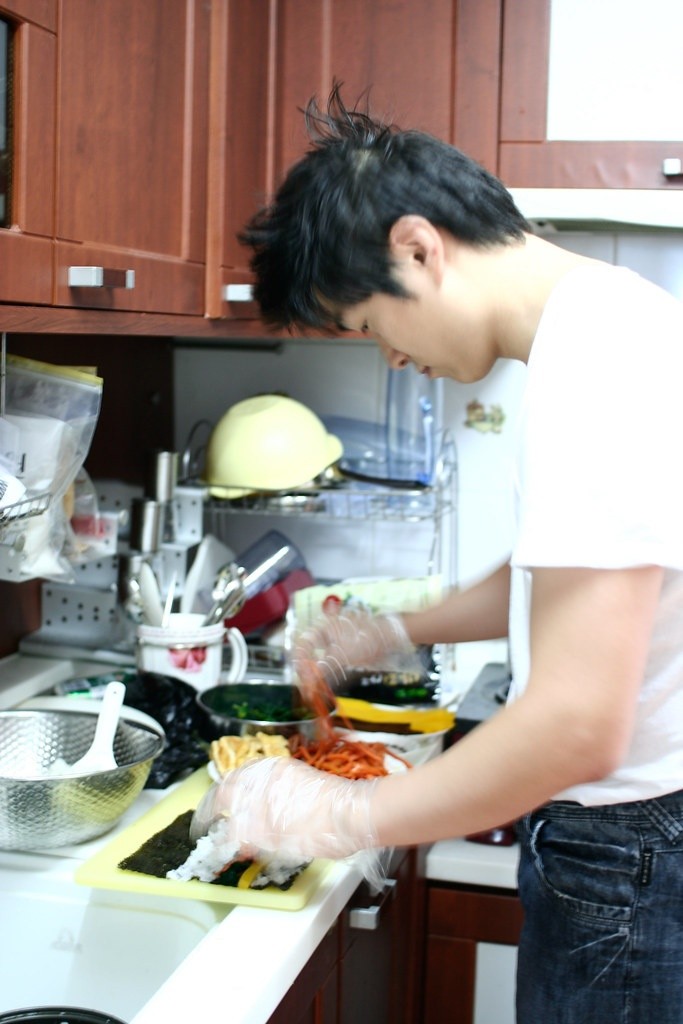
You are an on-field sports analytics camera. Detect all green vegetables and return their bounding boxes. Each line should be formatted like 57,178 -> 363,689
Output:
231,700 -> 316,721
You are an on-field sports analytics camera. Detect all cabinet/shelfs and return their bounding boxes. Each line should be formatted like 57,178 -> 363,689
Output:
417,836 -> 521,1024
0,0 -> 503,341
0,653 -> 457,1024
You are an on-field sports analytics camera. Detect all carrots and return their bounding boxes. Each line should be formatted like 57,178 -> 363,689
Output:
288,659 -> 412,781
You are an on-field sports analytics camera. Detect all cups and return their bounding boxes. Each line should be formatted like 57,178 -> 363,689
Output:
138,612 -> 247,691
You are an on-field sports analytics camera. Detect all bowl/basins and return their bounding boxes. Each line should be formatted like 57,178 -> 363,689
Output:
203,393 -> 342,499
195,682 -> 337,737
0,709 -> 167,851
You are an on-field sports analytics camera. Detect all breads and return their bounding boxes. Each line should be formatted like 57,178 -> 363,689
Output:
211,731 -> 290,781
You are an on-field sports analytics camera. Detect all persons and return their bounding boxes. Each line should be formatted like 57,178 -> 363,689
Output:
188,74 -> 683,1024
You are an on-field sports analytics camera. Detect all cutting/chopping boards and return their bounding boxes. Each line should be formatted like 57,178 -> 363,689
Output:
78,764 -> 334,911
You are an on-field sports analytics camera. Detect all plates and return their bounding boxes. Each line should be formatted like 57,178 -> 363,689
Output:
334,704 -> 449,748
